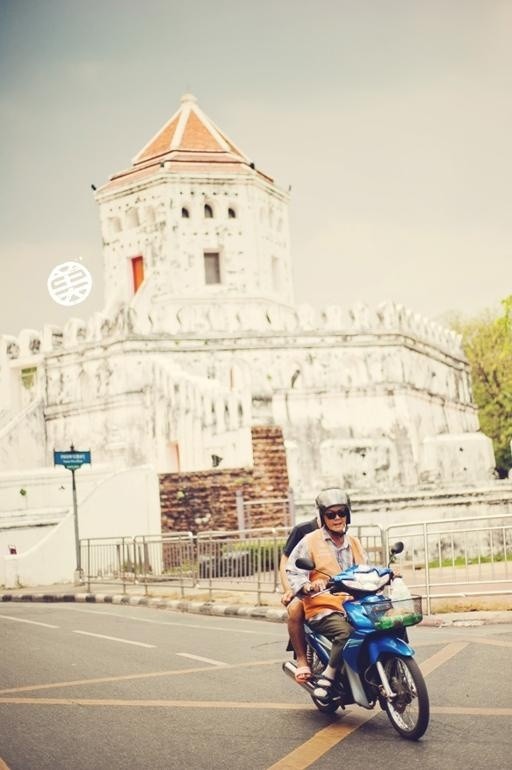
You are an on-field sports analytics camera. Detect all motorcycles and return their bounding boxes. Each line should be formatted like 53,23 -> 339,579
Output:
282,539 -> 436,742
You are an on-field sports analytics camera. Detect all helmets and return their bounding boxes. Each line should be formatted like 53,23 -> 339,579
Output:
315,487 -> 350,528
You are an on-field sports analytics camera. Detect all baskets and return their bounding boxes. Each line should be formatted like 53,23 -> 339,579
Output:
361,595 -> 422,631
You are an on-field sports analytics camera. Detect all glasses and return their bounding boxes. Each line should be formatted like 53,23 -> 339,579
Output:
326,511 -> 346,519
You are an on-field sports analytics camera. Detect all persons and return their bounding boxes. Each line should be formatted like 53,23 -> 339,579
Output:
282,487 -> 400,704
278,509 -> 327,684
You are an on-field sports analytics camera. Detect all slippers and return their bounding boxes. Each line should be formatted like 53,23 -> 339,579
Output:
295,667 -> 312,683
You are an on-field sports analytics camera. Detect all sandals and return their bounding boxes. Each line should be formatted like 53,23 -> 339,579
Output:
311,675 -> 334,699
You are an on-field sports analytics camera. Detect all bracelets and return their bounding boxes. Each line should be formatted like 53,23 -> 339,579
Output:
306,585 -> 311,595
